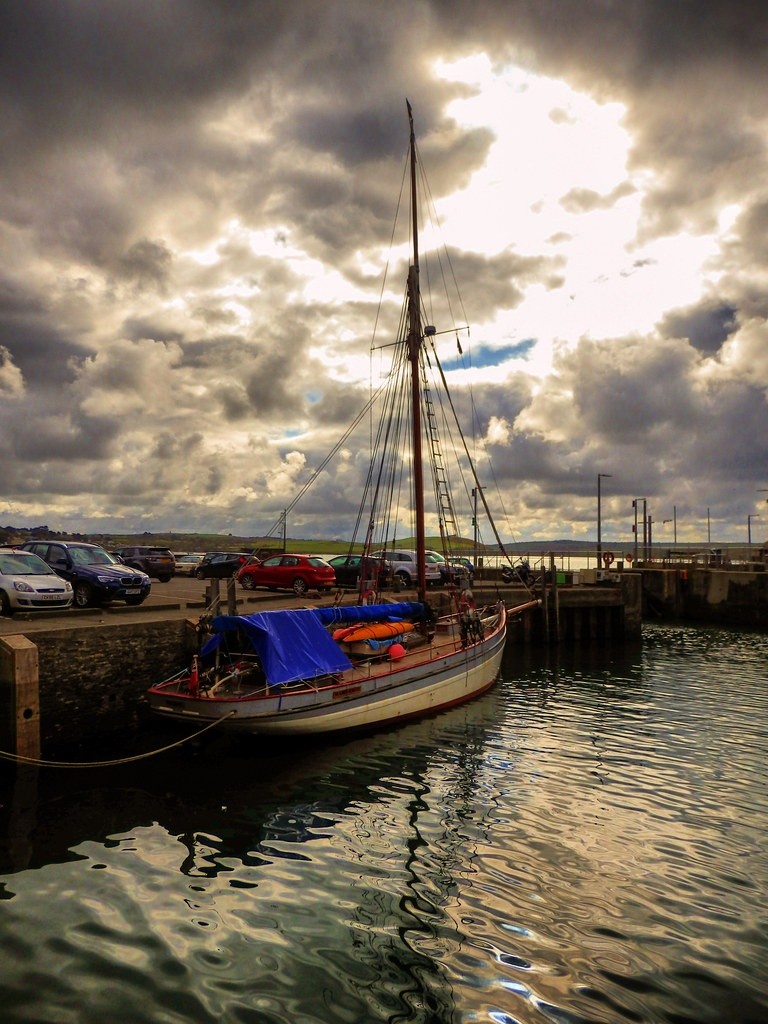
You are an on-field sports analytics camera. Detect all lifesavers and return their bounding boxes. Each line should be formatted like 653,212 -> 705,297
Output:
603,552 -> 614,564
363,590 -> 376,606
461,589 -> 475,615
626,554 -> 632,562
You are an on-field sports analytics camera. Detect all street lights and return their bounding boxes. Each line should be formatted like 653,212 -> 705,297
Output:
633,497 -> 648,568
747,513 -> 760,545
596,472 -> 613,567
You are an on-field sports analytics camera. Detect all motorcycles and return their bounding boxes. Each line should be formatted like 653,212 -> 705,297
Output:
500,556 -> 535,584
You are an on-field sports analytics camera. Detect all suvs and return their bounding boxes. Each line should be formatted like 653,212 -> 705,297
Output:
113,546 -> 176,583
21,540 -> 152,608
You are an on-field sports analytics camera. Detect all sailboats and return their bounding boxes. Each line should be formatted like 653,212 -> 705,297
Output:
130,97 -> 550,736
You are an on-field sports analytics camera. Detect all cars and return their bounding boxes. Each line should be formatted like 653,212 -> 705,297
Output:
323,555 -> 394,592
235,553 -> 336,593
174,551 -> 260,579
0,548 -> 74,617
367,547 -> 476,587
